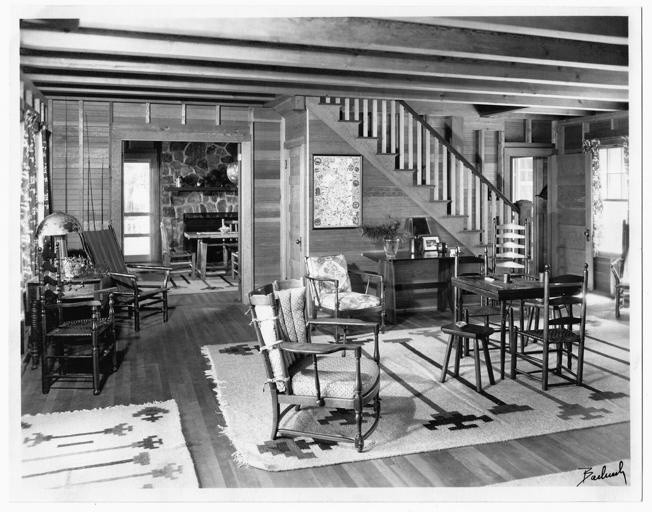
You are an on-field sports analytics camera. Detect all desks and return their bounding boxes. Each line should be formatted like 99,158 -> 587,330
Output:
361,250 -> 476,323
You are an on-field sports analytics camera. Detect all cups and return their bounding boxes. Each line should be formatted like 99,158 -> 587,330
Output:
437,242 -> 448,253
384,239 -> 399,259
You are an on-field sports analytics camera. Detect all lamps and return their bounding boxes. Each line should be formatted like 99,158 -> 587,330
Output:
36,213 -> 82,258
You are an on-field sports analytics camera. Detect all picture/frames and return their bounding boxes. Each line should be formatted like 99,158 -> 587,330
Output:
313,153 -> 363,229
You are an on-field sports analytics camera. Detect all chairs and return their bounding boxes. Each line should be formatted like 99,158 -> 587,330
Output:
76,225 -> 172,331
305,253 -> 385,357
609,220 -> 632,321
511,263 -> 589,391
231,252 -> 240,280
222,219 -> 239,265
245,278 -> 379,453
160,218 -> 195,288
38,242 -> 117,395
455,243 -> 514,356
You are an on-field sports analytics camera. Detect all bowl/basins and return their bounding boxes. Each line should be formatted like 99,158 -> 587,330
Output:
218,225 -> 231,234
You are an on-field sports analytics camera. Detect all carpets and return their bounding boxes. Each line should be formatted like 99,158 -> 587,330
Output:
202,315 -> 630,472
137,276 -> 239,294
21,399 -> 200,489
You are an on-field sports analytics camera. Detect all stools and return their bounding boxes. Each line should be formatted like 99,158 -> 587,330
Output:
524,301 -> 567,347
439,322 -> 496,392
